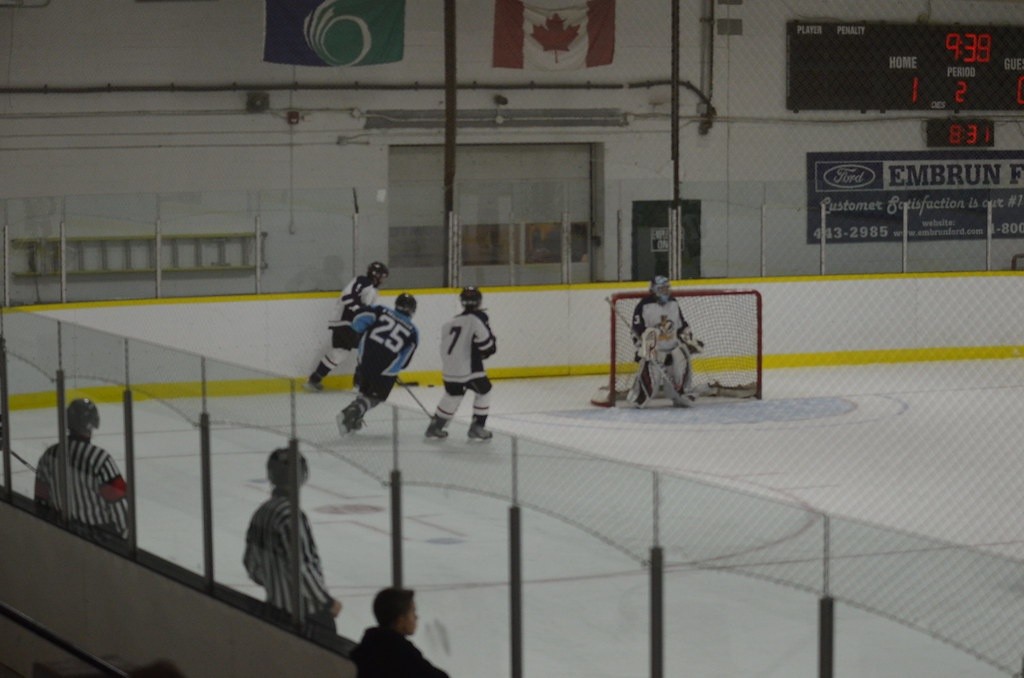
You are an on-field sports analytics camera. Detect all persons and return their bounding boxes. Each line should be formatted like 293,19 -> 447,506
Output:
35,399 -> 128,538
304,260 -> 389,392
627,275 -> 703,411
337,293 -> 418,436
245,448 -> 339,628
350,588 -> 448,678
424,286 -> 496,439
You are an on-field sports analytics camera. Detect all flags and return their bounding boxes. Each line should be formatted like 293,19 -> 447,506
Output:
267,0 -> 402,66
492,0 -> 616,69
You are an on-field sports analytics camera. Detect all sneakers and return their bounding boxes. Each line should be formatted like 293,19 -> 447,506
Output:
301,371 -> 323,391
466,414 -> 493,444
423,413 -> 448,443
335,392 -> 371,437
351,383 -> 360,394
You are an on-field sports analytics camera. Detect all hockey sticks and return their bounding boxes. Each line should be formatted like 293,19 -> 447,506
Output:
397,377 -> 434,418
395,375 -> 420,388
604,295 -> 696,408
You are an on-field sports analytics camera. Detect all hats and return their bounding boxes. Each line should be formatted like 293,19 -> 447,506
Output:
651,275 -> 673,291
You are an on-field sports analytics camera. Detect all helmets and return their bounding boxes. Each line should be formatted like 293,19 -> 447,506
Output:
395,293 -> 417,315
368,262 -> 389,287
67,398 -> 100,430
267,449 -> 308,486
460,287 -> 482,306
